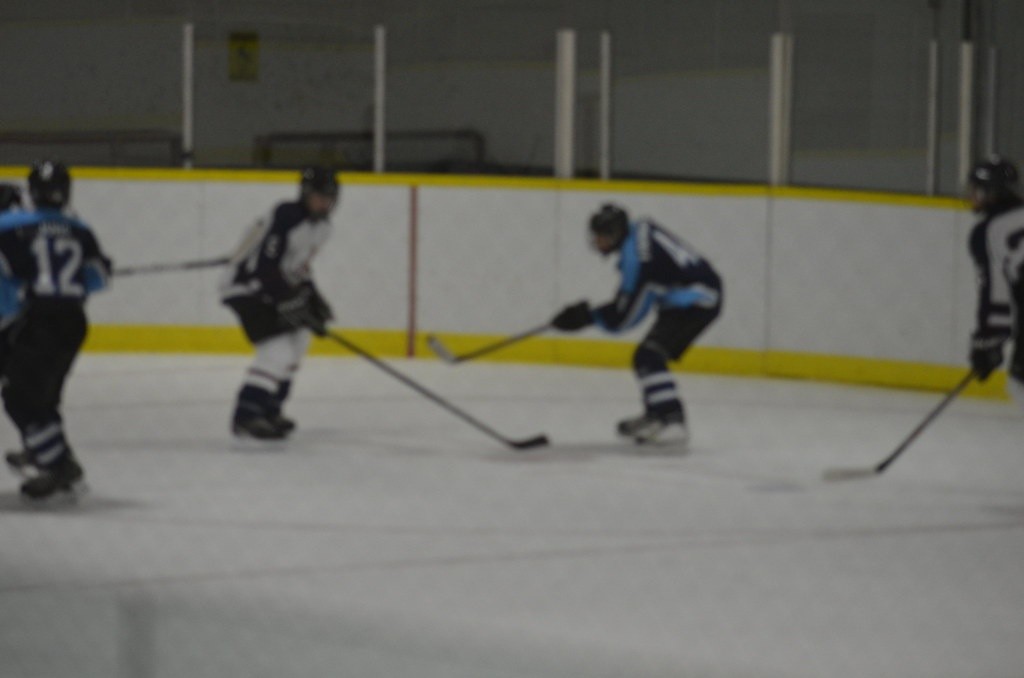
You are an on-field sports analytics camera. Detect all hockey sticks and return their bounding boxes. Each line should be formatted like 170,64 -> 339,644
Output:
424,321 -> 554,364
820,367 -> 978,481
326,332 -> 549,449
112,213 -> 275,278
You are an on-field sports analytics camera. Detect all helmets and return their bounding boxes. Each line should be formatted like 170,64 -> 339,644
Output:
965,158 -> 1019,216
299,168 -> 340,226
588,204 -> 630,255
28,154 -> 70,208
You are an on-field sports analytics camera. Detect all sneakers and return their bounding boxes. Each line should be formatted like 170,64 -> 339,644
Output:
617,382 -> 674,441
635,400 -> 687,453
258,385 -> 295,435
7,450 -> 45,477
16,462 -> 78,509
20,447 -> 92,493
232,385 -> 278,447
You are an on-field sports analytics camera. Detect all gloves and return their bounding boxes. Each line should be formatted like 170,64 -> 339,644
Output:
552,301 -> 594,333
596,290 -> 634,331
273,286 -> 326,340
970,325 -> 1010,381
295,280 -> 333,321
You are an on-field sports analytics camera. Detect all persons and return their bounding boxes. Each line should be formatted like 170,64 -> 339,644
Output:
552,203 -> 724,447
963,150 -> 1024,388
223,158 -> 340,442
1,160 -> 117,498
